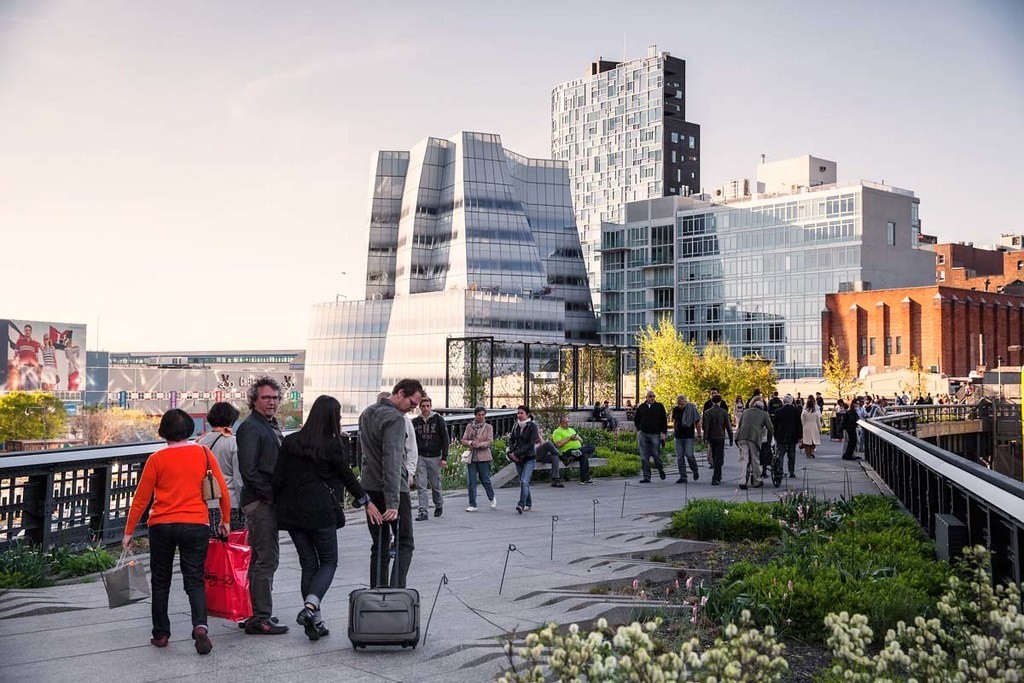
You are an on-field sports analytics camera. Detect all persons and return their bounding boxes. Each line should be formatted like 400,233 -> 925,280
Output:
593,385 -> 960,490
196,401 -> 247,540
375,391 -> 419,559
552,415 -> 596,485
13,324 -> 39,391
460,406 -> 497,512
412,396 -> 449,522
234,374 -> 291,636
357,378 -> 428,589
120,406 -> 233,655
37,333 -> 57,390
528,414 -> 574,488
506,405 -> 539,515
276,394 -> 387,642
63,337 -> 81,392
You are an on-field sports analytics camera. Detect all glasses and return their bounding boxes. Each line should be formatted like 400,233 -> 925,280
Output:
25,328 -> 30,331
44,338 -> 48,341
648,395 -> 655,398
408,396 -> 418,409
258,395 -> 280,401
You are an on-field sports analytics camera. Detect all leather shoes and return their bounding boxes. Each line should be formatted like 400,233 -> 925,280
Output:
238,616 -> 280,629
245,618 -> 290,635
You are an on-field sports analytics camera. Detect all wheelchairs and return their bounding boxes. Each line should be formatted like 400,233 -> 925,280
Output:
745,440 -> 784,488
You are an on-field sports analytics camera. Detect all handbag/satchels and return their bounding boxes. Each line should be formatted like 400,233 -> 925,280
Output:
106,547 -> 150,610
328,488 -> 346,529
202,444 -> 223,500
203,533 -> 254,623
461,451 -> 473,464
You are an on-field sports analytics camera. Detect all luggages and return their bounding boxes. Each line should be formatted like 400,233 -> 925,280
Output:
348,514 -> 421,651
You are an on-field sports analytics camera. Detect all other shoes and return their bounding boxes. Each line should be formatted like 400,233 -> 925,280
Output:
676,477 -> 687,483
639,478 -> 651,483
192,627 -> 212,655
434,506 -> 443,517
558,454 -> 574,467
487,496 -> 497,508
658,468 -> 666,480
707,434 -> 868,491
579,479 -> 595,485
523,505 -> 532,512
571,450 -> 582,458
550,477 -> 564,487
415,512 -> 428,521
296,608 -> 319,641
693,470 -> 699,480
309,620 -> 329,641
150,633 -> 169,647
389,542 -> 396,558
465,506 -> 478,512
516,506 -> 523,514
